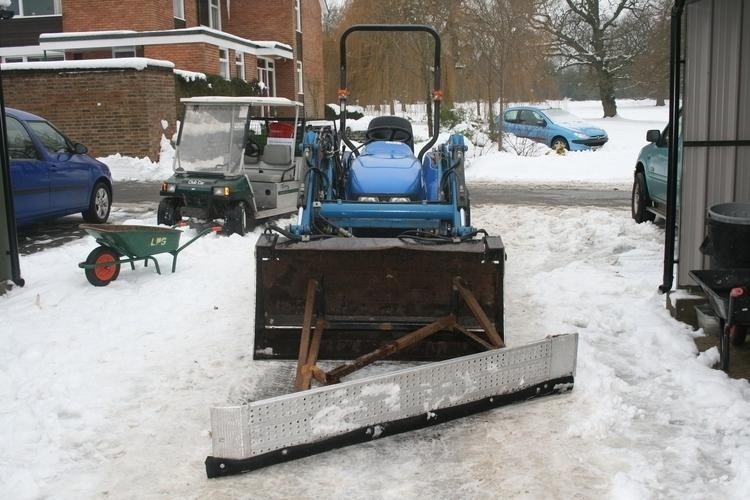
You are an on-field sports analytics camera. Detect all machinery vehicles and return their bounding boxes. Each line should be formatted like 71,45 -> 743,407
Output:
254,24 -> 505,364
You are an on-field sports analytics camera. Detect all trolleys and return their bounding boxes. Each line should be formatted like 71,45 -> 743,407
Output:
79,223 -> 222,287
689,269 -> 750,371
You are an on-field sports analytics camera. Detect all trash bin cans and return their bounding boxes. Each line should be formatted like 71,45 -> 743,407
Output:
699,201 -> 750,269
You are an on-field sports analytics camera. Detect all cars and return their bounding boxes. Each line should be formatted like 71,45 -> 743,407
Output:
488,104 -> 609,152
631,108 -> 683,225
2,107 -> 113,224
